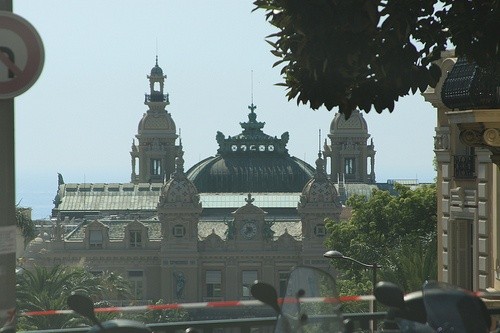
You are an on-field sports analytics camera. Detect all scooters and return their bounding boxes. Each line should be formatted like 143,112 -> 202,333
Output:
250,264 -> 500,333
66,290 -> 204,333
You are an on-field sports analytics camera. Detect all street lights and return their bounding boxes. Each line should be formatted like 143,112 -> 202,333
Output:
323,251 -> 384,332
